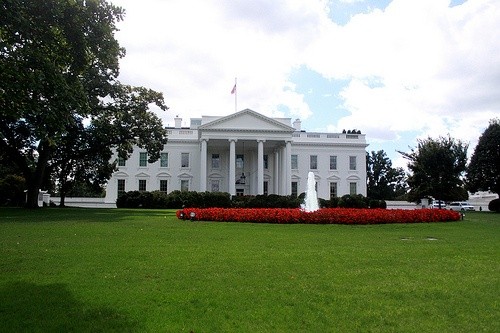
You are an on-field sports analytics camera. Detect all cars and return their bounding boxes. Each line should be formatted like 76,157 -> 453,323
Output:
446,202 -> 474,212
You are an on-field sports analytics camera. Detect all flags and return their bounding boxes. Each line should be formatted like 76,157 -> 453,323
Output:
231,83 -> 236,94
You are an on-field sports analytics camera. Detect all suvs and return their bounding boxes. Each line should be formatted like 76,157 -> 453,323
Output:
430,200 -> 446,209
